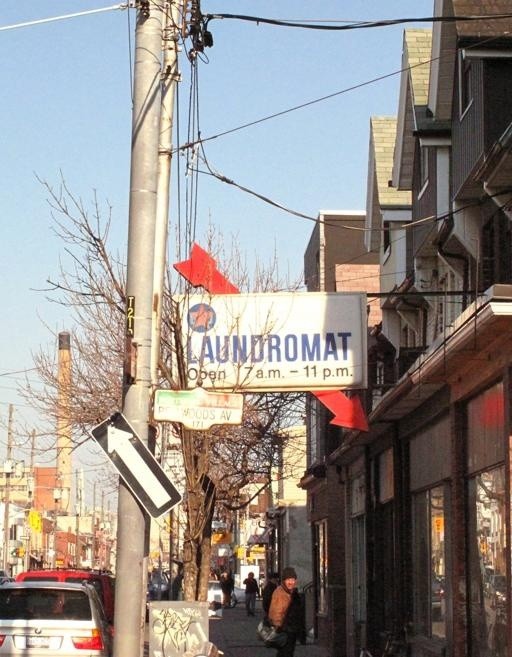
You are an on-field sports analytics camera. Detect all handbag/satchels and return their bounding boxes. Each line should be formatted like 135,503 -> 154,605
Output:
257,620 -> 287,648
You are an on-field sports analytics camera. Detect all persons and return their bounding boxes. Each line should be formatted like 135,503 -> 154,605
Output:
243,572 -> 260,617
262,567 -> 307,657
219,571 -> 234,607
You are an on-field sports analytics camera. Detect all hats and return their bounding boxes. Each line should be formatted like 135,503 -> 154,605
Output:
282,567 -> 296,580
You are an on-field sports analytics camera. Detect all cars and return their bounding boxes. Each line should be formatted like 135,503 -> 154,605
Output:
208,580 -> 248,607
1,566 -> 171,655
